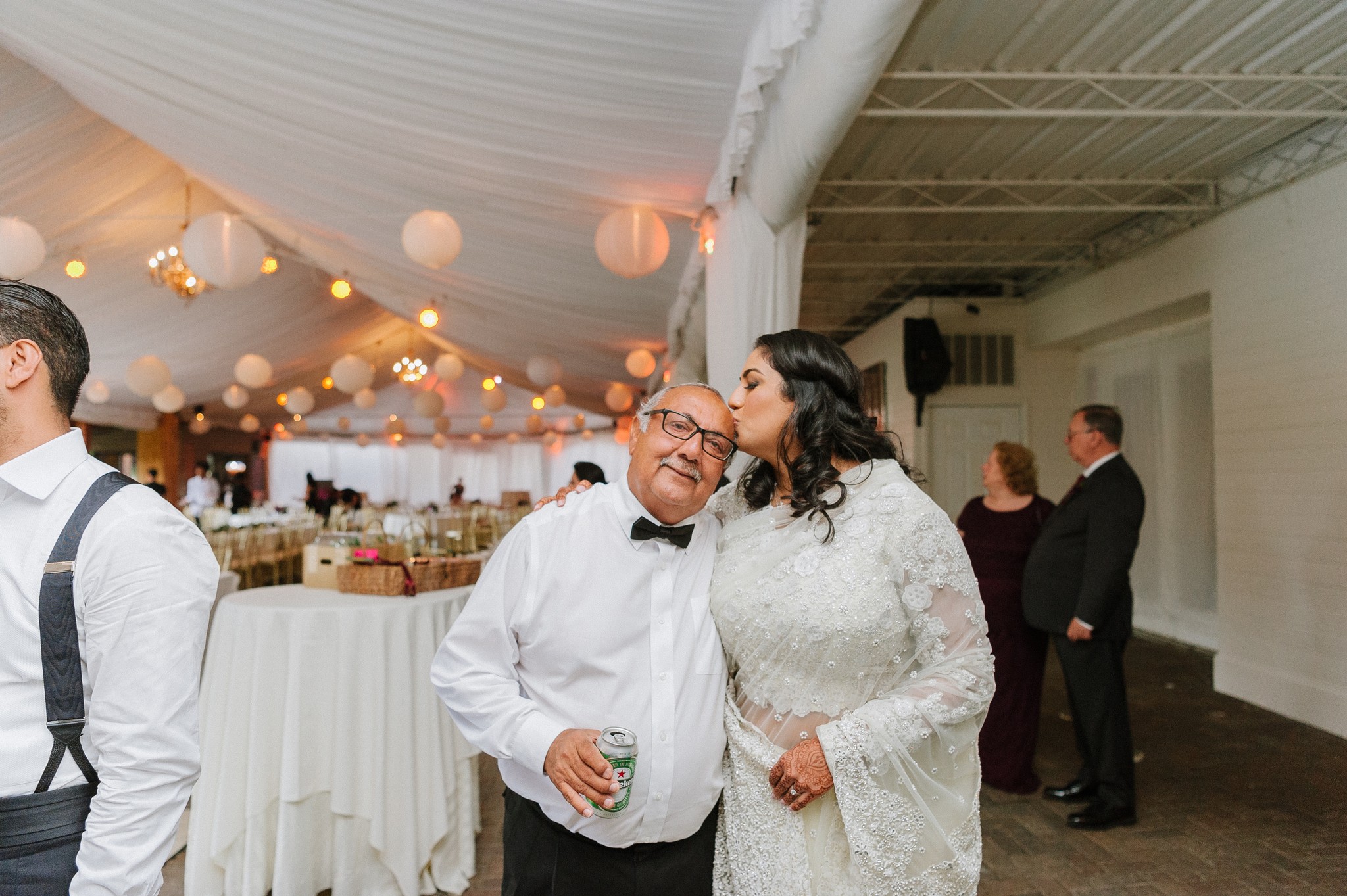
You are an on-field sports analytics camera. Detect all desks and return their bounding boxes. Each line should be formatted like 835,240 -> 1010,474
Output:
182,582 -> 485,896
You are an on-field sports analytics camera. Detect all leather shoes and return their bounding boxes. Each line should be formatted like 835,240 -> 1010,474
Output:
1044,780 -> 1096,799
1067,802 -> 1135,829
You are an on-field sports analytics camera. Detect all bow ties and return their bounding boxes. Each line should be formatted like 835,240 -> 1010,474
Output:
630,516 -> 696,549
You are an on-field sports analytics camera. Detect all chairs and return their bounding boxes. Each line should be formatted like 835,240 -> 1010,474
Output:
202,502 -> 533,590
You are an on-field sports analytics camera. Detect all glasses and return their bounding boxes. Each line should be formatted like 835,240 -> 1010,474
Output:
644,409 -> 737,461
1066,429 -> 1095,438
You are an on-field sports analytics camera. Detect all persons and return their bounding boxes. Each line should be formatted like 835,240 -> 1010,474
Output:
945,435 -> 1061,794
1017,398 -> 1148,836
569,461 -> 606,490
425,382 -> 738,896
174,460 -> 222,531
0,271 -> 220,896
324,489 -> 365,527
294,471 -> 320,511
532,323 -> 997,895
222,472 -> 253,515
142,467 -> 168,499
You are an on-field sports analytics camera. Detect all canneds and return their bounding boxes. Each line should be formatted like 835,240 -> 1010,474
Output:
584,727 -> 638,819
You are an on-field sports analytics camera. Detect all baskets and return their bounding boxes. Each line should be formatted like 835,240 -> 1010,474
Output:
338,557 -> 441,594
429,555 -> 481,588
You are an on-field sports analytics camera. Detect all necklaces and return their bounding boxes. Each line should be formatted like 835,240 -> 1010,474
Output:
773,457 -> 842,511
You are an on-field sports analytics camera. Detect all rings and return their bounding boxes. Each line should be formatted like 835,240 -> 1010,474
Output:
790,784 -> 802,796
